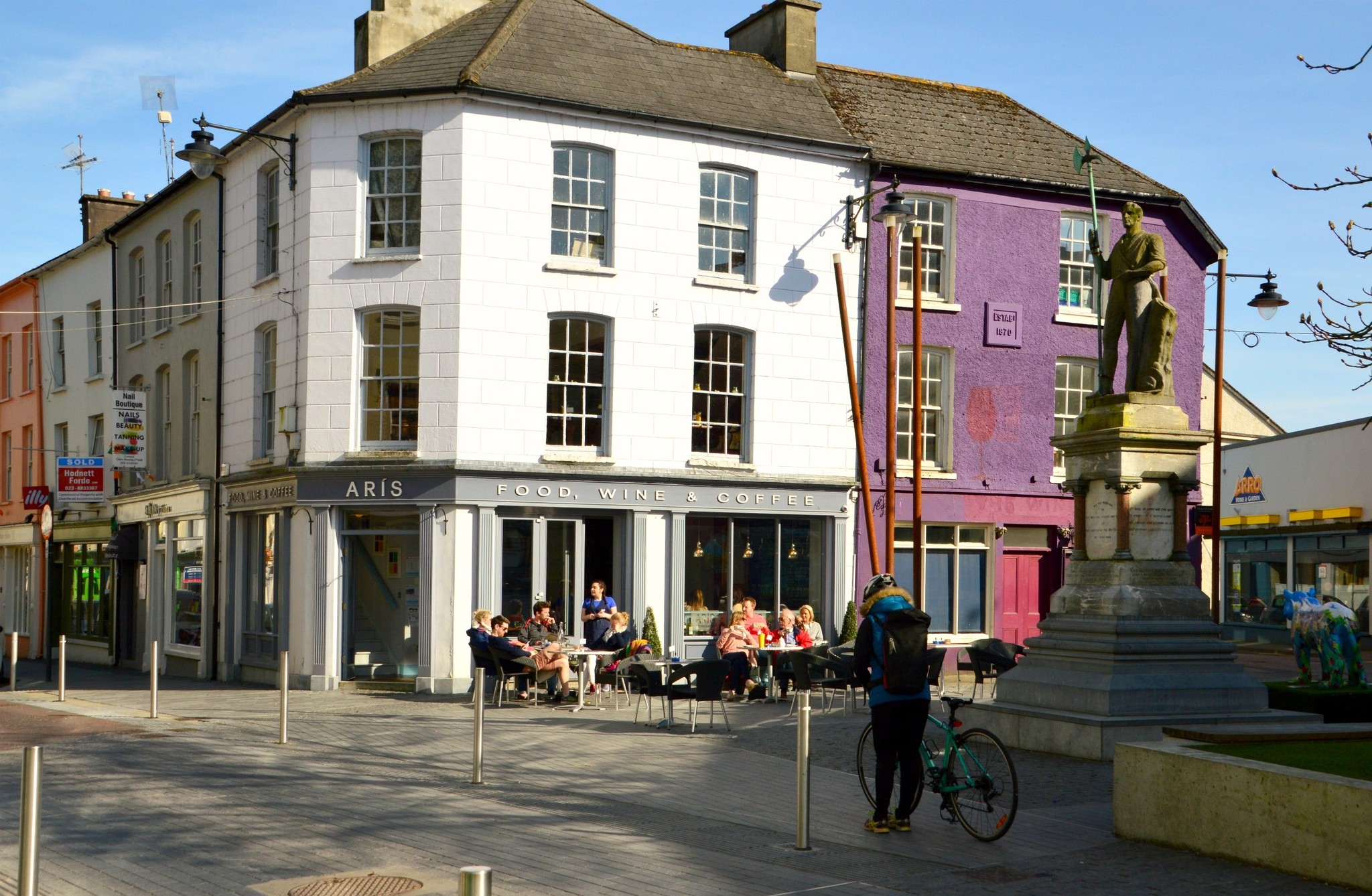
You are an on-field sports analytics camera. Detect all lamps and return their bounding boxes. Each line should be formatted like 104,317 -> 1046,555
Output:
25,513 -> 59,524
838,173 -> 918,247
58,509 -> 99,521
1204,267 -> 1289,321
175,112 -> 299,191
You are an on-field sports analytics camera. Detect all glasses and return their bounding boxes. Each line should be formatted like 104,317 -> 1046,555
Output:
739,617 -> 747,622
497,624 -> 507,631
778,617 -> 791,621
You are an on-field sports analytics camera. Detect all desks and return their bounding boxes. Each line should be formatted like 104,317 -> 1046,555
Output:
842,651 -> 872,715
787,639 -> 829,646
926,643 -> 974,697
630,661 -> 697,729
546,649 -> 620,712
737,646 -> 803,704
531,645 -> 590,702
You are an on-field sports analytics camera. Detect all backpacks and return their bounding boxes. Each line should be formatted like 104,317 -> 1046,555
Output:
872,608 -> 932,694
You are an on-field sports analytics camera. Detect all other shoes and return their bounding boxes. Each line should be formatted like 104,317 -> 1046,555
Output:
735,692 -> 745,699
726,692 -> 734,701
746,683 -> 760,693
518,693 -> 534,701
791,685 -> 797,692
601,683 -> 610,692
779,696 -> 788,703
589,686 -> 596,696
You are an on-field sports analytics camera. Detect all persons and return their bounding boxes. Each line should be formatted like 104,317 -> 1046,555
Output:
685,588 -> 710,610
466,609 -> 530,700
578,612 -> 632,697
487,615 -> 579,705
720,586 -> 744,612
580,580 -> 617,692
1087,202 -> 1166,397
853,574 -> 935,833
715,596 -> 824,701
518,601 -> 559,696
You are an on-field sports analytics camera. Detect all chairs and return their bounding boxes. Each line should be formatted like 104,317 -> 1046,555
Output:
469,635 -> 1027,736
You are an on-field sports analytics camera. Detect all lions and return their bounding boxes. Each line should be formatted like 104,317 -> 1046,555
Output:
1282,587 -> 1368,688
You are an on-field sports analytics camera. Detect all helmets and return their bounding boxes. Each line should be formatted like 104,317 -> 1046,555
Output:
862,574 -> 896,602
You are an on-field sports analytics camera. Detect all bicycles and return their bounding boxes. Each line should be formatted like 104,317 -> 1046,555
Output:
856,689 -> 1019,842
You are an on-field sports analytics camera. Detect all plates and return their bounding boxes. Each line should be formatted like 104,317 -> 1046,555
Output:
785,645 -> 798,647
742,645 -> 754,647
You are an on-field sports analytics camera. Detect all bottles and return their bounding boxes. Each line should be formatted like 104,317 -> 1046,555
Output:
779,636 -> 784,647
557,622 -> 565,644
684,617 -> 693,636
711,618 -> 718,636
719,617 -> 725,636
766,616 -> 771,631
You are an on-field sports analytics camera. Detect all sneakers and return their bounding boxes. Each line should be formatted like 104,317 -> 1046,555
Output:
863,809 -> 890,832
567,655 -> 579,665
561,694 -> 579,704
888,805 -> 912,831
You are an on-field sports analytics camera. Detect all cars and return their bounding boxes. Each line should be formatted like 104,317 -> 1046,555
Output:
1260,593 -> 1369,633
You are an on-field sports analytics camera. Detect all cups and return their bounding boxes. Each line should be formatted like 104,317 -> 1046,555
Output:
733,625 -> 742,630
758,634 -> 765,648
784,627 -> 793,633
541,639 -> 570,648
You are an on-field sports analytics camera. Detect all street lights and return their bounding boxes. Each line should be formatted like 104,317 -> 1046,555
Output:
1206,249 -> 1289,626
